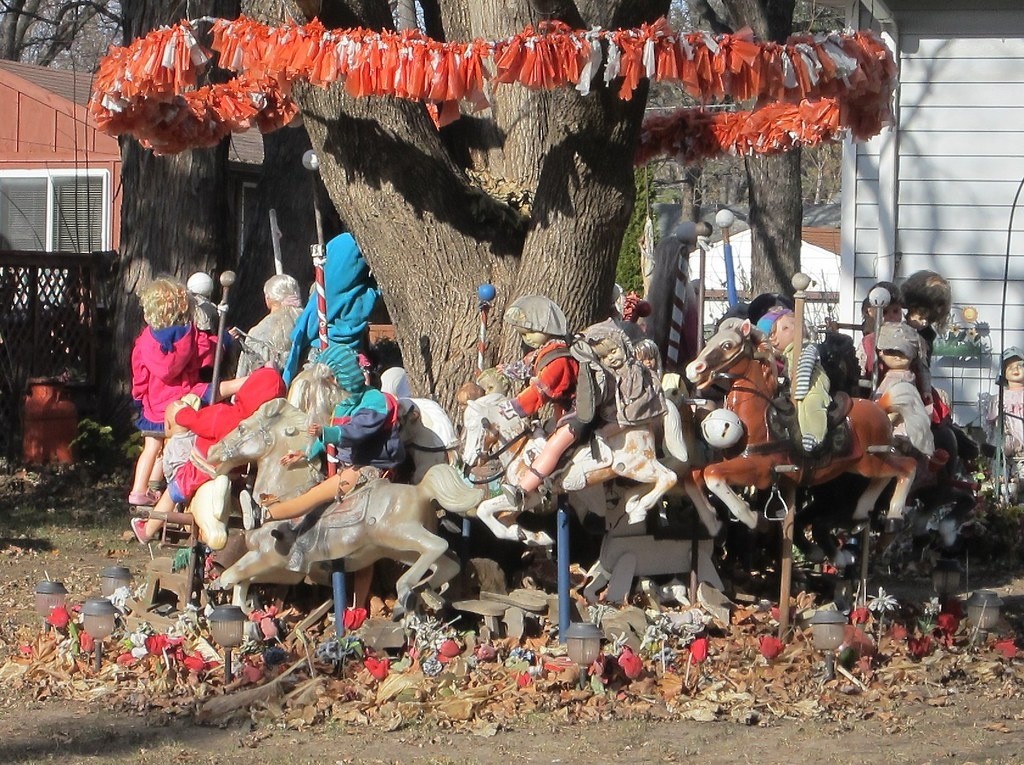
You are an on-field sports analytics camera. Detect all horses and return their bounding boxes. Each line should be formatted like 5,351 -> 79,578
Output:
203,359 -> 487,623
575,471 -> 732,613
790,419 -> 1014,572
685,315 -> 938,538
460,388 -> 692,554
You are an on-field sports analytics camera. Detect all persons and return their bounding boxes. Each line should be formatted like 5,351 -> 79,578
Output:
129,233 -> 379,546
240,346 -> 407,529
474,270 -> 952,505
986,345 -> 1024,459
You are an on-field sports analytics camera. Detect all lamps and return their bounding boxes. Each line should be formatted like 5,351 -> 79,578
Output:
77,598 -> 121,640
31,579 -> 71,619
207,604 -> 249,648
809,610 -> 850,651
561,621 -> 608,667
962,589 -> 1006,631
98,565 -> 136,598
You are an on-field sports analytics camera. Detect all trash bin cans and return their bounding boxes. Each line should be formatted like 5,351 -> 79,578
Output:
21,377 -> 95,465
